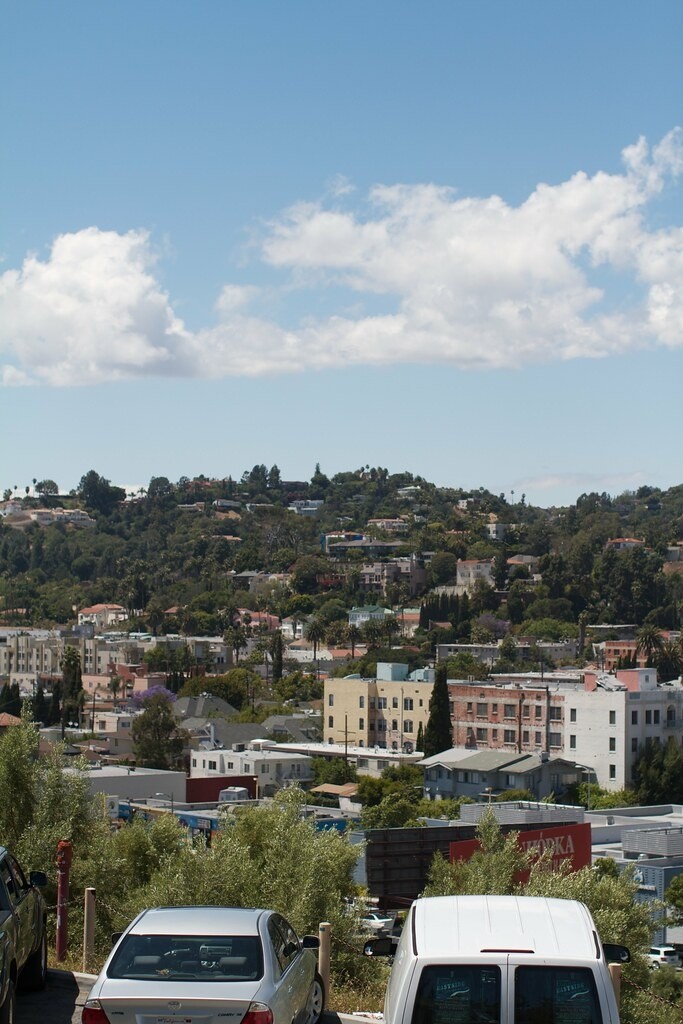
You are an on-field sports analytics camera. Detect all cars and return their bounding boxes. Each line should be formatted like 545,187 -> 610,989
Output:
639,947 -> 680,972
361,910 -> 394,930
82,905 -> 330,1024
0,845 -> 50,1024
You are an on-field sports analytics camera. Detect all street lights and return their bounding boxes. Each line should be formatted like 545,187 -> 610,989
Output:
155,792 -> 174,817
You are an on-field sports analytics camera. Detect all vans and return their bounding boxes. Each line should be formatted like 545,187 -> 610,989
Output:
363,891 -> 633,1024
